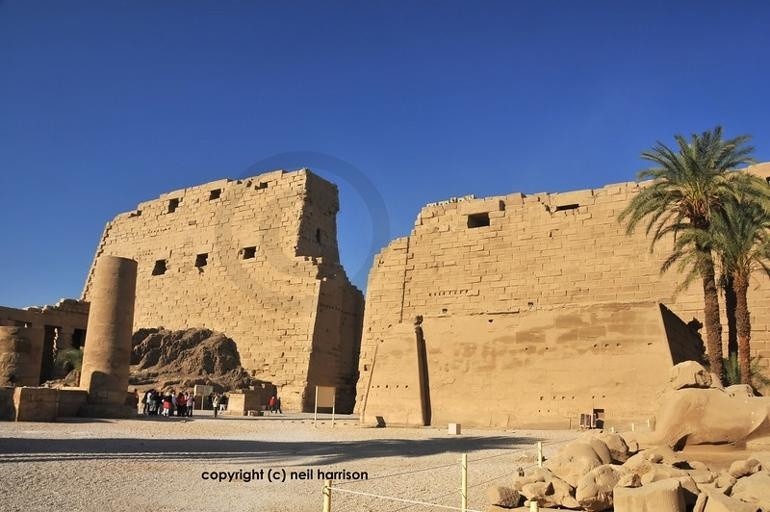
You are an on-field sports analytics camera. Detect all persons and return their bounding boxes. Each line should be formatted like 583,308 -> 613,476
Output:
135,388 -> 139,408
142,389 -> 194,418
269,395 -> 276,413
209,391 -> 227,417
275,397 -> 283,414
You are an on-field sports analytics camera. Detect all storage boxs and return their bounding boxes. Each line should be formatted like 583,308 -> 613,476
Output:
447,423 -> 463,435
